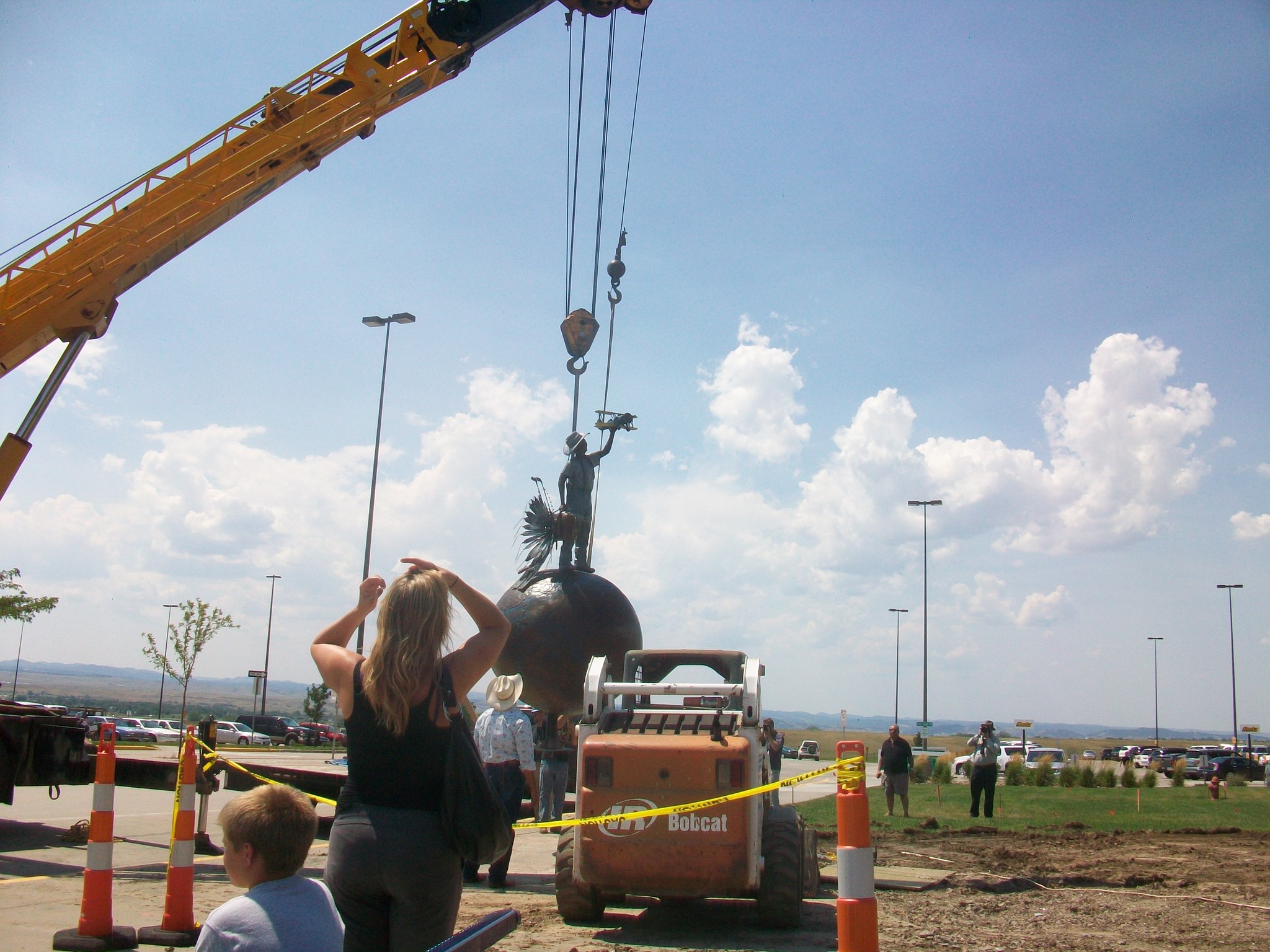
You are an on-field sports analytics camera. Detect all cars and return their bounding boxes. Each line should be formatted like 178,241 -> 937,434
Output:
1083,750 -> 1096,760
215,721 -> 271,746
951,740 -> 1072,778
14,699 -> 201,742
1100,743 -> 1270,790
781,746 -> 798,759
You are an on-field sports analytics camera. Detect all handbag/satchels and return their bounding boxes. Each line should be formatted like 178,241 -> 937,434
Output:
438,657 -> 510,865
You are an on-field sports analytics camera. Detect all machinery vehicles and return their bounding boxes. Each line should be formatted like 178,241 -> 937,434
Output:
551,646 -> 823,930
0,0 -> 663,572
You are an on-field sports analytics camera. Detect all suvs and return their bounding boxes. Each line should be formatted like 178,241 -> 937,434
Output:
798,739 -> 820,762
234,714 -> 347,748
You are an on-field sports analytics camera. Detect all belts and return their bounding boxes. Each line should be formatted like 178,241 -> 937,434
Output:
975,762 -> 995,768
483,759 -> 520,768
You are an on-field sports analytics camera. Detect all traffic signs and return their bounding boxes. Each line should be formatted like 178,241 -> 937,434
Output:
248,670 -> 267,678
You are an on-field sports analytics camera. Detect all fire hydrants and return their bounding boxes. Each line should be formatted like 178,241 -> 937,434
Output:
1207,776 -> 1220,800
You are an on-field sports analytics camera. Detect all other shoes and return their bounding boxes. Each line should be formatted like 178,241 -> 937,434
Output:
884,812 -> 894,816
486,878 -> 515,887
904,814 -> 909,817
550,827 -> 561,834
539,827 -> 548,833
464,875 -> 487,884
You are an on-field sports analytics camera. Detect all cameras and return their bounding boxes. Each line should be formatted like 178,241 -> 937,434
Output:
763,724 -> 769,731
980,726 -> 989,733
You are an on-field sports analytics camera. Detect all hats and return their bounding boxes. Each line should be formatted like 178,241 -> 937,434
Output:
981,720 -> 996,731
486,673 -> 523,710
531,707 -> 540,713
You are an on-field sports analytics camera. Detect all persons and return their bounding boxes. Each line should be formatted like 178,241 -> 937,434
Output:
1120,752 -> 1130,770
463,673 -> 538,889
876,725 -> 914,817
527,708 -> 577,833
192,781 -> 346,952
764,718 -> 784,805
967,720 -> 1000,817
558,428 -> 617,572
310,558 -> 510,952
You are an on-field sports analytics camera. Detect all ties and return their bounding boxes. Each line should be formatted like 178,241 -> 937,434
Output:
980,738 -> 986,754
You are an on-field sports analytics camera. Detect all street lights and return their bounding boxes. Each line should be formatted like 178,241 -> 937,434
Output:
260,575 -> 282,716
908,499 -> 943,752
157,604 -> 178,720
1217,584 -> 1243,757
888,608 -> 908,724
354,311 -> 417,657
1147,637 -> 1164,747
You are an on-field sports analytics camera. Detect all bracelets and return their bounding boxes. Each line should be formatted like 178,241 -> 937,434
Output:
448,576 -> 459,589
910,768 -> 913,770
